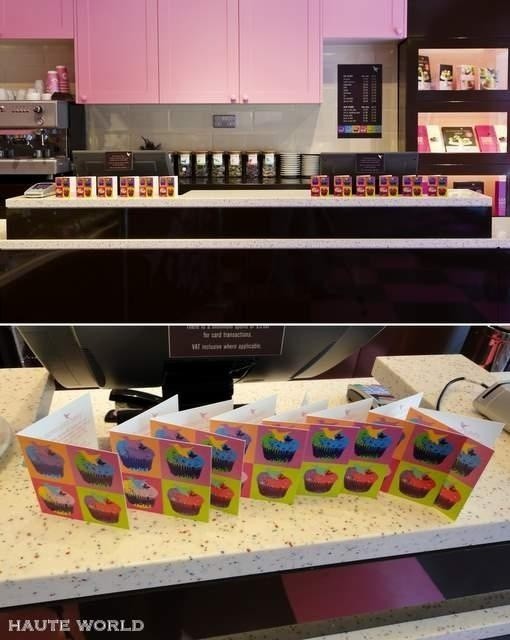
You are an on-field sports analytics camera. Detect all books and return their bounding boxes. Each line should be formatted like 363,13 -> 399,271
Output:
309,172 -> 449,198
54,175 -> 179,199
13,390 -> 504,530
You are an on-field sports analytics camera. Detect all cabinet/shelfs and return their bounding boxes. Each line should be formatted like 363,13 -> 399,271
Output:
158,0 -> 323,105
73,0 -> 158,106
321,0 -> 407,46
398,0 -> 510,175
0,0 -> 73,39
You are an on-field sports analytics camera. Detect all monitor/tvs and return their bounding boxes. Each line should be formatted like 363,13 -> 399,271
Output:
72,150 -> 174,195
17,326 -> 386,412
320,152 -> 419,194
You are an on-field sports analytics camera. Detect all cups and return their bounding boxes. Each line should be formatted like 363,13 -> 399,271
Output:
0,65 -> 70,101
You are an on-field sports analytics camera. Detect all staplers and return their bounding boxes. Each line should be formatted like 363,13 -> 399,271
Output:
105,389 -> 163,422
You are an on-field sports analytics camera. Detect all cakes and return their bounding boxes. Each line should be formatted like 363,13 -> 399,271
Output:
26,427 -> 480,523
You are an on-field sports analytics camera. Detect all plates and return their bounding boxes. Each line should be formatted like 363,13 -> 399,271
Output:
279,153 -> 320,180
